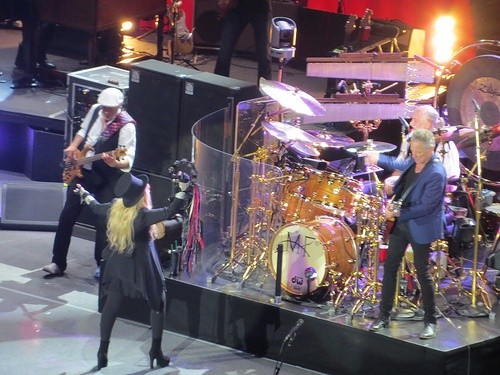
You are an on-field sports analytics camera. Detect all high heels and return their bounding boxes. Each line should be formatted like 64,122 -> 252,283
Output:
97,350 -> 109,370
149,344 -> 170,369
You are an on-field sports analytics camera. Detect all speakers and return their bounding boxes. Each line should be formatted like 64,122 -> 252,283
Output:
64,60 -> 262,265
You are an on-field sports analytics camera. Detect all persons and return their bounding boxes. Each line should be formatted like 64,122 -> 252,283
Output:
213,0 -> 271,87
43,86 -> 136,278
14,0 -> 58,70
72,172 -> 193,371
357,103 -> 460,339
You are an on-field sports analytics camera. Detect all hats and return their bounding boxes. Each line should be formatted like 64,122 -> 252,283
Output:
114,173 -> 149,207
96,88 -> 123,107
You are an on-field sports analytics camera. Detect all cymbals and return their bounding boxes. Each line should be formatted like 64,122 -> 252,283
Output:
260,79 -> 326,116
259,120 -> 321,150
306,129 -> 355,148
343,140 -> 398,154
433,127 -> 474,143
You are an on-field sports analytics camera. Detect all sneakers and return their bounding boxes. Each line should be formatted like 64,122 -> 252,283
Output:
42,262 -> 62,275
94,266 -> 100,277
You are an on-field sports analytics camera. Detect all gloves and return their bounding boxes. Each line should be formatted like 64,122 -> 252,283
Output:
73,184 -> 90,198
176,171 -> 190,192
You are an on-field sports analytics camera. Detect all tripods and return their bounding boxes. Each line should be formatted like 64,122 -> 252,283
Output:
225,110 -> 500,320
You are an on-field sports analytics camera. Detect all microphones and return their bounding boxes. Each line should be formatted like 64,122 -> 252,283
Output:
472,99 -> 481,111
399,116 -> 411,130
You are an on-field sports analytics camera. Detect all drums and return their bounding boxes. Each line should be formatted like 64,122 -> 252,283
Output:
268,215 -> 357,296
268,165 -> 342,235
310,170 -> 362,216
274,142 -> 320,170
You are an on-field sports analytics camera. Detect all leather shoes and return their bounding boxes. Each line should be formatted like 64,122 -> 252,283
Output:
368,318 -> 391,330
419,323 -> 437,339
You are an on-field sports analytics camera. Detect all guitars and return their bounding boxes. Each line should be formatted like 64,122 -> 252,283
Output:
64,145 -> 127,184
382,194 -> 403,241
